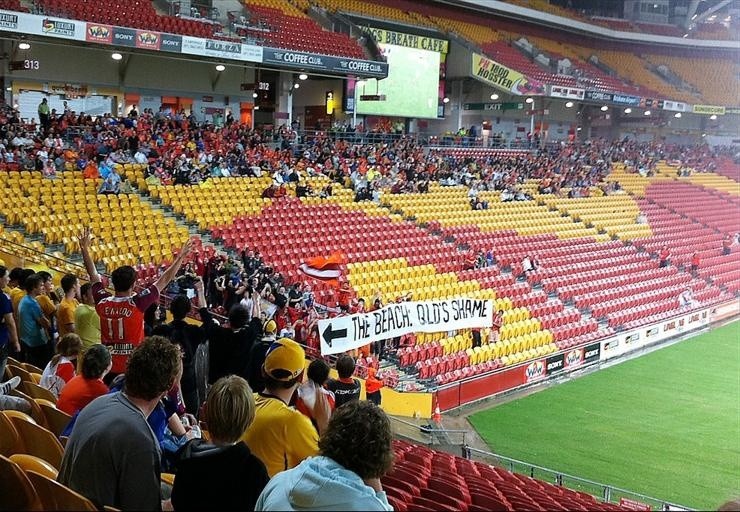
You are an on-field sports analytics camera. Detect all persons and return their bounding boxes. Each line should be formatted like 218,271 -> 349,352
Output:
657,244 -> 673,268
470,327 -> 483,349
677,289 -> 691,307
722,232 -> 740,255
691,253 -> 700,277
488,308 -> 505,344
288,0 -> 342,15
462,242 -> 498,270
359,33 -> 369,45
686,286 -> 694,306
636,212 -> 647,224
510,251 -> 540,281
189,3 -> 269,45
72,227 -> 193,385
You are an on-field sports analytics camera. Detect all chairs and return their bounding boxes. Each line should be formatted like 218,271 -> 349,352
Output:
1,356 -> 119,512
0,147 -> 740,391
380,438 -> 652,512
160,472 -> 175,486
441,0 -> 737,107
3,1 -> 441,60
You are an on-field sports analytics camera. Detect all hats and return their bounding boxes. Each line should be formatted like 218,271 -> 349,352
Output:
263,337 -> 306,381
